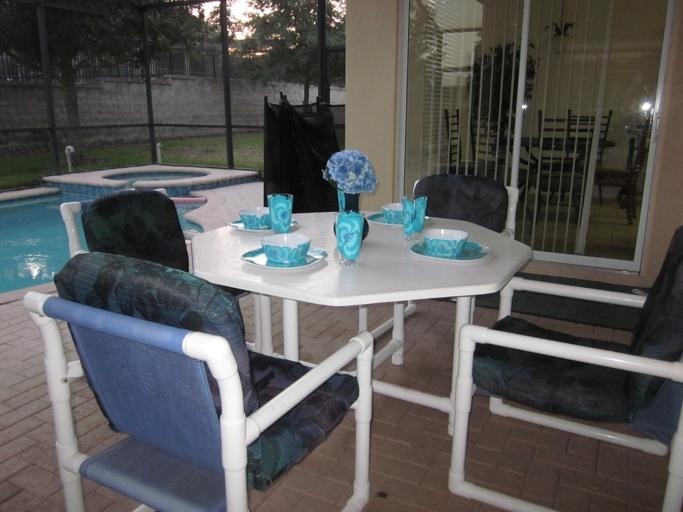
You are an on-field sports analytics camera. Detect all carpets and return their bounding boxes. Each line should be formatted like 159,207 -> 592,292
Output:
431,271 -> 653,332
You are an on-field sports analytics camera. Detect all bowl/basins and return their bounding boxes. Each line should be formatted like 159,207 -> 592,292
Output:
236,206 -> 272,229
380,201 -> 406,224
260,233 -> 313,264
421,226 -> 470,258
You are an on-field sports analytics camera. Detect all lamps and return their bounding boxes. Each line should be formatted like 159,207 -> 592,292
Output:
543,0 -> 577,57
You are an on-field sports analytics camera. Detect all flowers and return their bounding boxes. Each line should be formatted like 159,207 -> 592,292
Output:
319,148 -> 380,196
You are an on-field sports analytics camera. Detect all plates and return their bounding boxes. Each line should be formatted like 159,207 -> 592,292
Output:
366,212 -> 431,229
409,239 -> 491,263
228,217 -> 299,234
241,246 -> 328,272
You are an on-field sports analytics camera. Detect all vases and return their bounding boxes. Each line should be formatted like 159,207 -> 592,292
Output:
332,192 -> 369,248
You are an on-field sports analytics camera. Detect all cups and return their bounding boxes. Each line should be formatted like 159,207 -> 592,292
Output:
335,184 -> 346,213
401,193 -> 430,240
267,190 -> 294,236
333,209 -> 365,267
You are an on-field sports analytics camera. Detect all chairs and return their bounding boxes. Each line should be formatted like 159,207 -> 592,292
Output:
388,172 -> 521,367
442,108 -> 650,237
22,250 -> 374,511
447,224 -> 682,512
59,186 -> 260,352
